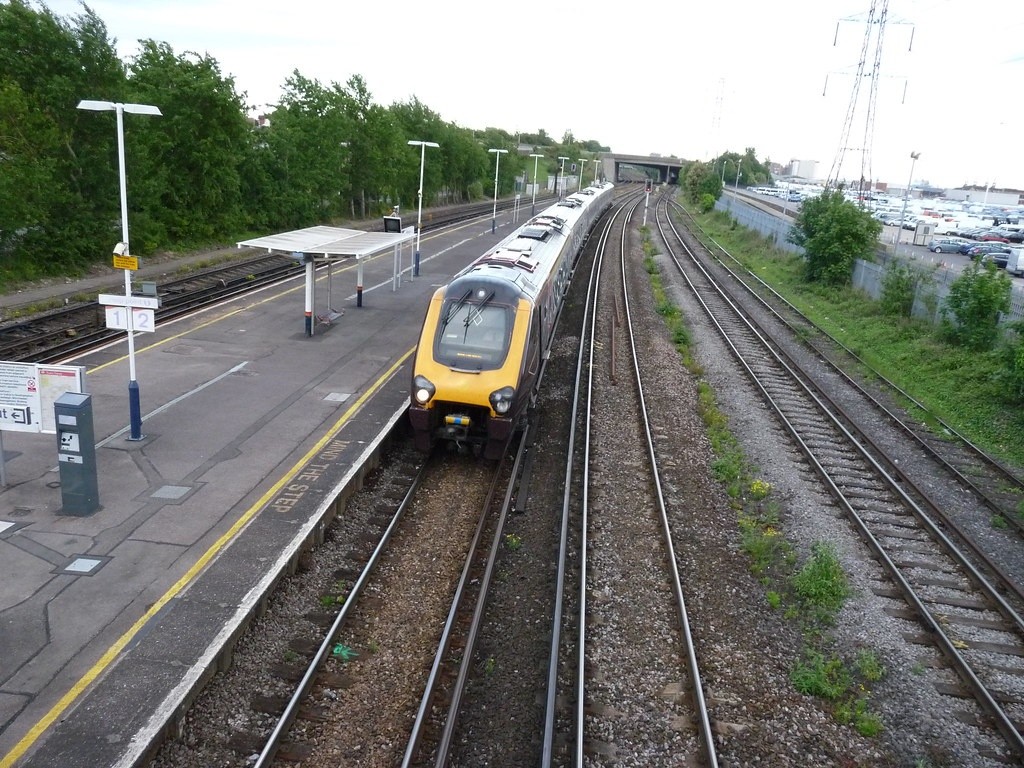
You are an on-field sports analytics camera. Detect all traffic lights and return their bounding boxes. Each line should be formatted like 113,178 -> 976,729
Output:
644,178 -> 652,193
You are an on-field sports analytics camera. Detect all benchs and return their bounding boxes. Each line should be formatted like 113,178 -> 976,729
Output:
315,308 -> 344,329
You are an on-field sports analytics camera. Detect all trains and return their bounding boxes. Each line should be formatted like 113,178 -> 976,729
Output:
408,177 -> 615,460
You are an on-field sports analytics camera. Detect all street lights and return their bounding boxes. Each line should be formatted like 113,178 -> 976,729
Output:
720,160 -> 728,186
736,159 -> 743,194
529,154 -> 546,218
488,149 -> 509,234
408,140 -> 439,278
893,150 -> 921,252
712,160 -> 716,173
76,100 -> 163,441
577,159 -> 588,192
558,157 -> 569,199
592,159 -> 601,186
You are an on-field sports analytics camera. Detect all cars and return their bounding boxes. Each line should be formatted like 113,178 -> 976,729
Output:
747,180 -> 1024,277
